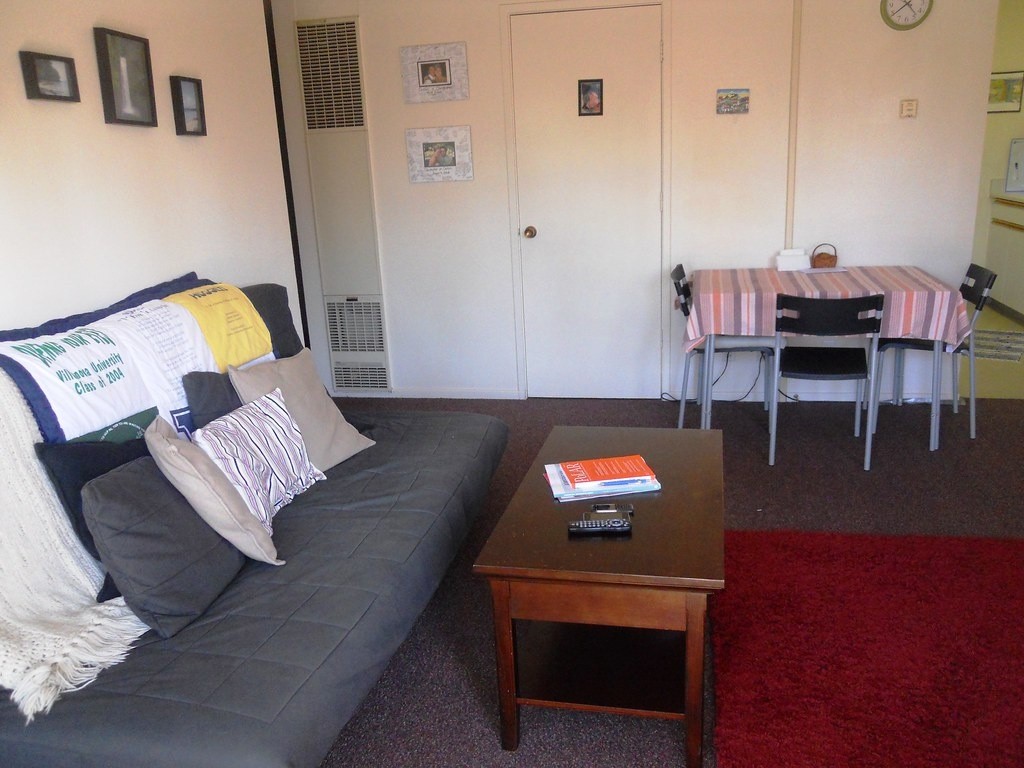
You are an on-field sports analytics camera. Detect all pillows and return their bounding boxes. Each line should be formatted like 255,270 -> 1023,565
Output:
31,347 -> 376,636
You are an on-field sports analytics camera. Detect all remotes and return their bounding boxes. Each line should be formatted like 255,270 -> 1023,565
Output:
568,519 -> 632,535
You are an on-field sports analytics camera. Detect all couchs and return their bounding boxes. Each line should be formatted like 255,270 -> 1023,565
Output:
0,270 -> 506,767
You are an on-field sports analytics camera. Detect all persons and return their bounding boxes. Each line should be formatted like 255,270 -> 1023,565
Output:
428,145 -> 454,167
424,64 -> 447,84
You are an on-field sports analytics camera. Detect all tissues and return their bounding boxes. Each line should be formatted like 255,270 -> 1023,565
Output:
775,248 -> 811,272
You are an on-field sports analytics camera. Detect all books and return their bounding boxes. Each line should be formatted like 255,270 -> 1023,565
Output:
544,453 -> 661,503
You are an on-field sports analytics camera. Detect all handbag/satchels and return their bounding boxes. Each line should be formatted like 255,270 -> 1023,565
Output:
811,243 -> 837,267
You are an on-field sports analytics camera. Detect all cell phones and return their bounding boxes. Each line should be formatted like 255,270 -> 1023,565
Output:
583,512 -> 630,521
595,504 -> 634,515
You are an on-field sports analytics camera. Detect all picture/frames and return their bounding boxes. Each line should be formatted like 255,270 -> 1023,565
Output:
986,71 -> 1024,112
18,51 -> 81,102
170,76 -> 206,135
578,80 -> 603,116
406,125 -> 473,183
92,26 -> 158,127
398,41 -> 470,103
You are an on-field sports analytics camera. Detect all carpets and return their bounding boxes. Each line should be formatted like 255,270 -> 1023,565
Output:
710,529 -> 1024,768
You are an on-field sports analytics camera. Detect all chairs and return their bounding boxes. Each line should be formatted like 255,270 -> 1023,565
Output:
672,264 -> 996,470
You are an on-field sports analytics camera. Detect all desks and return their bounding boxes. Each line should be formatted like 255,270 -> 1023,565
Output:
472,426 -> 726,767
683,266 -> 974,451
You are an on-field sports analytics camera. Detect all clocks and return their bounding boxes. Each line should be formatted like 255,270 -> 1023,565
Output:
880,0 -> 935,32
